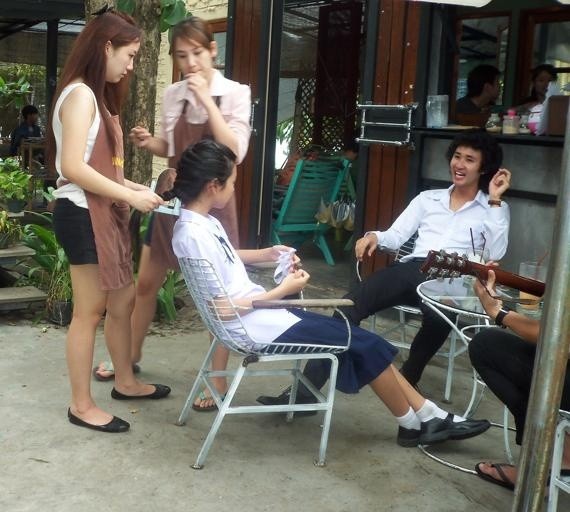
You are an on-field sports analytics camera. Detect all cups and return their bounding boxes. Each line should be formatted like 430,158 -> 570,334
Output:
519,261 -> 546,310
426,95 -> 449,128
462,247 -> 489,283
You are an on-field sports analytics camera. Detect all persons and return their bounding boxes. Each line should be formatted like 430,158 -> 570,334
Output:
260,133 -> 511,417
8,104 -> 41,157
522,65 -> 558,109
50,11 -> 172,433
161,138 -> 491,448
452,63 -> 501,127
91,16 -> 254,412
467,234 -> 568,498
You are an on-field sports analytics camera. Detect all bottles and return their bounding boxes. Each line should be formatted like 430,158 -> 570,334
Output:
485,113 -> 503,133
529,112 -> 540,135
502,110 -> 518,134
519,115 -> 532,134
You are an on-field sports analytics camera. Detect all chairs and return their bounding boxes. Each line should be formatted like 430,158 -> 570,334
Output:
174,256 -> 351,470
271,156 -> 350,267
356,229 -> 489,405
546,409 -> 570,512
271,150 -> 321,210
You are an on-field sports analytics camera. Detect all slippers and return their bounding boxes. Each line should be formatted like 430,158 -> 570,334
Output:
475,459 -> 519,493
191,385 -> 228,412
93,359 -> 141,381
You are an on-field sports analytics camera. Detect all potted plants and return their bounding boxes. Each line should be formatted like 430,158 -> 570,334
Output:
43,265 -> 72,326
0,208 -> 23,249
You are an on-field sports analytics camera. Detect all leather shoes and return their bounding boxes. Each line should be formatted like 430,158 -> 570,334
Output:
256,389 -> 318,416
111,381 -> 172,401
418,412 -> 491,445
68,404 -> 130,432
397,424 -> 422,448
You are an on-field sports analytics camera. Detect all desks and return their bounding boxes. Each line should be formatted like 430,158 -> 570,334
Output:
20,138 -> 57,216
416,277 -> 544,476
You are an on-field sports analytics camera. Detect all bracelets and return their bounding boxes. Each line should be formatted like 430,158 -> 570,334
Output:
487,197 -> 504,209
495,301 -> 511,330
